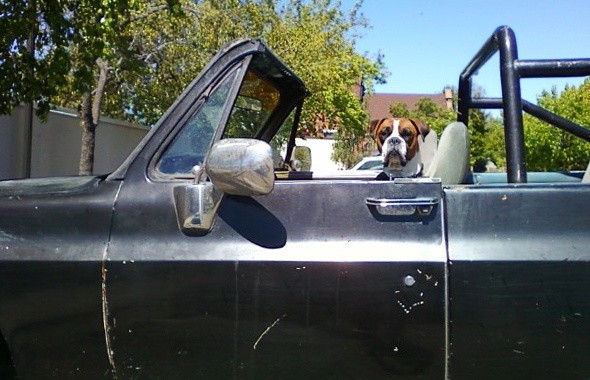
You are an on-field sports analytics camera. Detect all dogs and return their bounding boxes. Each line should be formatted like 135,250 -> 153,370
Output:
367,117 -> 431,181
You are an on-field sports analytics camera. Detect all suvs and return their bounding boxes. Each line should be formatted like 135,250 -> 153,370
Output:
0,27 -> 590,380
